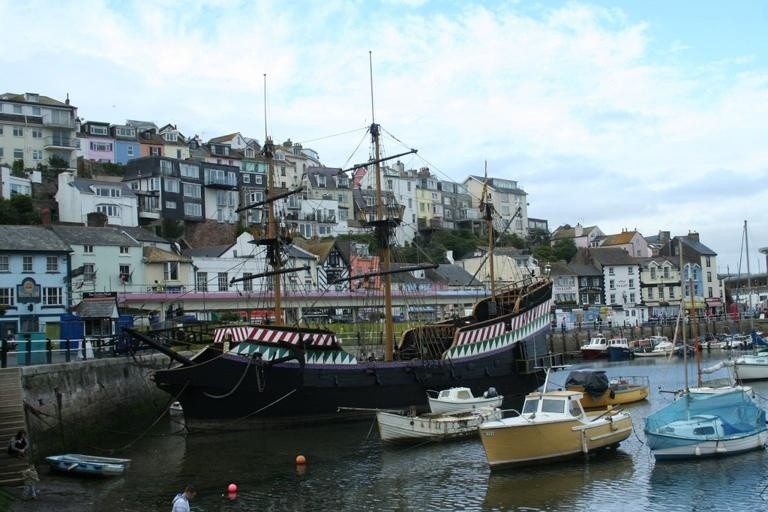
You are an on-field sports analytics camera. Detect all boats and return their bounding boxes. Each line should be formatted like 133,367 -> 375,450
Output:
422,384 -> 506,412
41,452 -> 133,478
364,409 -> 507,448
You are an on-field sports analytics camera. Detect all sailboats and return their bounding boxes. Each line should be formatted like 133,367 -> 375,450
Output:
109,47 -> 560,418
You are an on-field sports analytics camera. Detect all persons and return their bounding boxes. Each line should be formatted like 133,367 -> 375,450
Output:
20,463 -> 41,501
170,484 -> 198,512
8,428 -> 30,457
